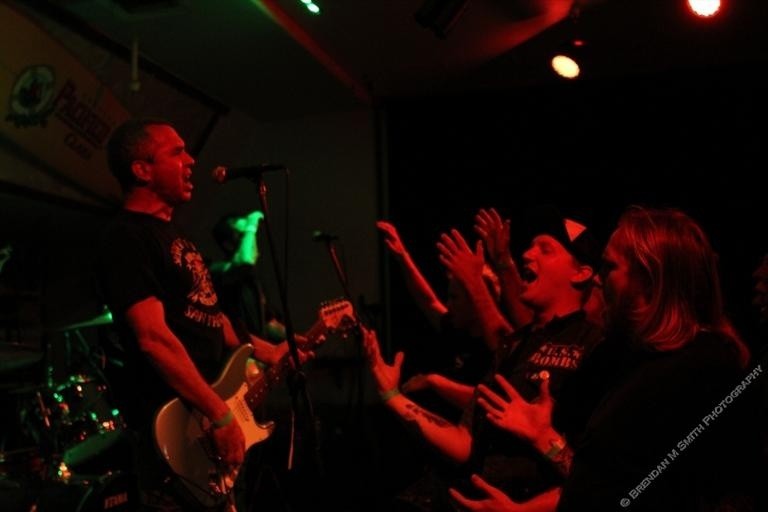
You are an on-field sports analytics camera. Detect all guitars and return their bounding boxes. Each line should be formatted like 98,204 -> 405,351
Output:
155,297 -> 357,494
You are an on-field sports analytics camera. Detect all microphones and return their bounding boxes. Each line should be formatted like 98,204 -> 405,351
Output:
310,227 -> 341,242
212,161 -> 288,183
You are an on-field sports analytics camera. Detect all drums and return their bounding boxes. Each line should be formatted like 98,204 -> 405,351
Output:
45,373 -> 125,468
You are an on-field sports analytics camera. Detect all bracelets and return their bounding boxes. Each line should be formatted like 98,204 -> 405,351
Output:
213,409 -> 234,429
244,224 -> 259,233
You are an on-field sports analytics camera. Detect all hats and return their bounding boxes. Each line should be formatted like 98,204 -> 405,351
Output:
532,217 -> 601,270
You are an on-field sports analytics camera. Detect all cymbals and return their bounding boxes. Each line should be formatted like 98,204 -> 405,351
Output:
0,348 -> 43,366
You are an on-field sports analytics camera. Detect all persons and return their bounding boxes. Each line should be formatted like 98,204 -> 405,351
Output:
97,121 -> 325,512
206,210 -> 286,341
